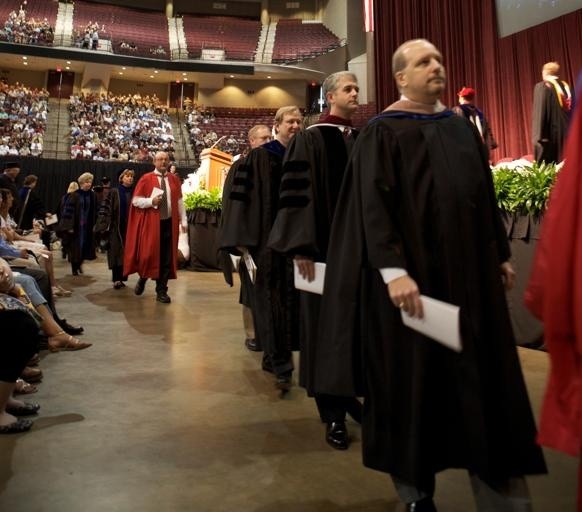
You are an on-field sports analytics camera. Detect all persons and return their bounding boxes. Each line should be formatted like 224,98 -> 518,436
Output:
58,151 -> 188,303
0,160 -> 92,431
311,37 -> 551,512
451,87 -> 498,165
524,84 -> 582,512
531,61 -> 576,169
222,72 -> 367,450
1,80 -> 246,163
0,5 -> 168,56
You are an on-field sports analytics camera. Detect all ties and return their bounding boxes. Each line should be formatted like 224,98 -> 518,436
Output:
160,174 -> 168,220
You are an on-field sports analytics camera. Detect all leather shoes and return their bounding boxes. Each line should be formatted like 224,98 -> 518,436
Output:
245,338 -> 263,353
325,414 -> 351,451
404,498 -> 439,512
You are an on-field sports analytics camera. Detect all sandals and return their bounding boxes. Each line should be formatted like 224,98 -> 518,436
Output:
51,285 -> 73,298
47,331 -> 93,354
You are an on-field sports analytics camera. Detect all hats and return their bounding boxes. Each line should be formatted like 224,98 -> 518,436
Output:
457,87 -> 476,100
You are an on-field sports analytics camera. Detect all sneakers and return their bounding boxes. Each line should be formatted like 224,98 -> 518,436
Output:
73,265 -> 84,276
156,292 -> 171,303
14,351 -> 43,395
274,375 -> 293,390
0,418 -> 35,434
134,278 -> 148,296
6,402 -> 42,416
58,318 -> 84,335
113,280 -> 126,290
